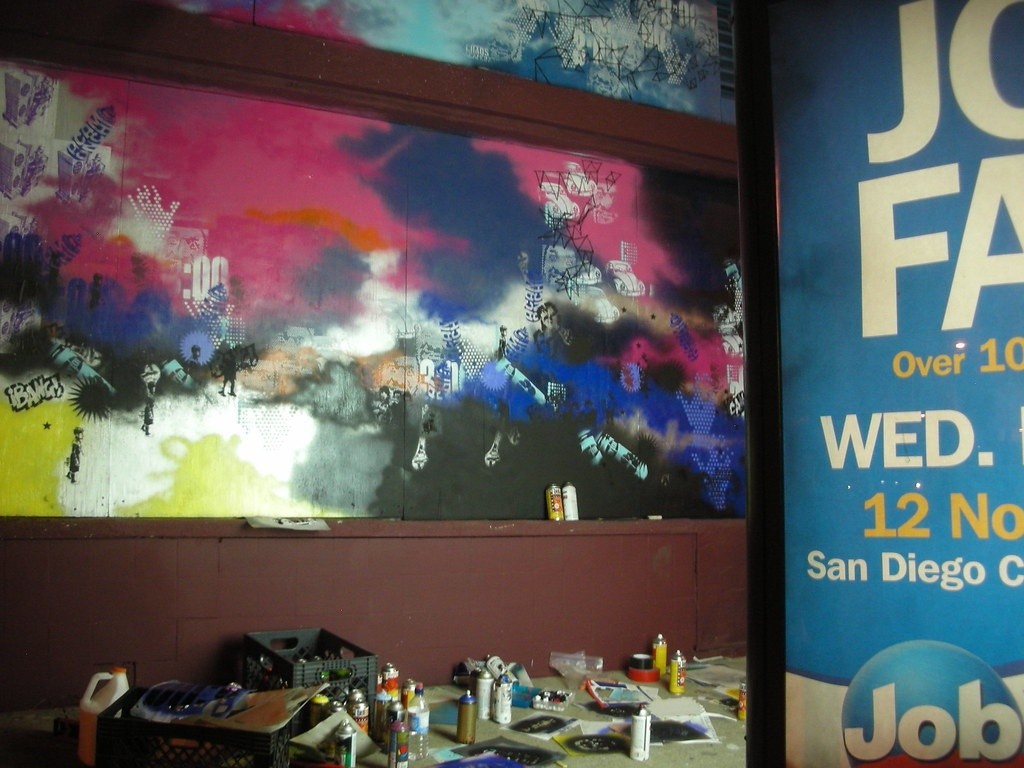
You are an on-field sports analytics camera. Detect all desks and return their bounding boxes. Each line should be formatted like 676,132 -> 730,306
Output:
0,657 -> 746,768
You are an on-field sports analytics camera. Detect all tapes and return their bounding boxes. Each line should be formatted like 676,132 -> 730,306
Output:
629,653 -> 654,670
627,667 -> 660,682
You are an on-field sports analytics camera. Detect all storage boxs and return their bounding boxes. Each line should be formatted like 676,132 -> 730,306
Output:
241,627 -> 378,741
95,685 -> 292,768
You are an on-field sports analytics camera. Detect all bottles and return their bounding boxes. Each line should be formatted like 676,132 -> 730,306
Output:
652,633 -> 667,675
669,650 -> 687,695
486,654 -> 518,685
311,663 -> 429,768
630,706 -> 651,762
737,676 -> 747,720
78,667 -> 130,765
546,483 -> 564,521
457,690 -> 478,744
475,670 -> 495,721
562,482 -> 579,521
492,670 -> 513,724
